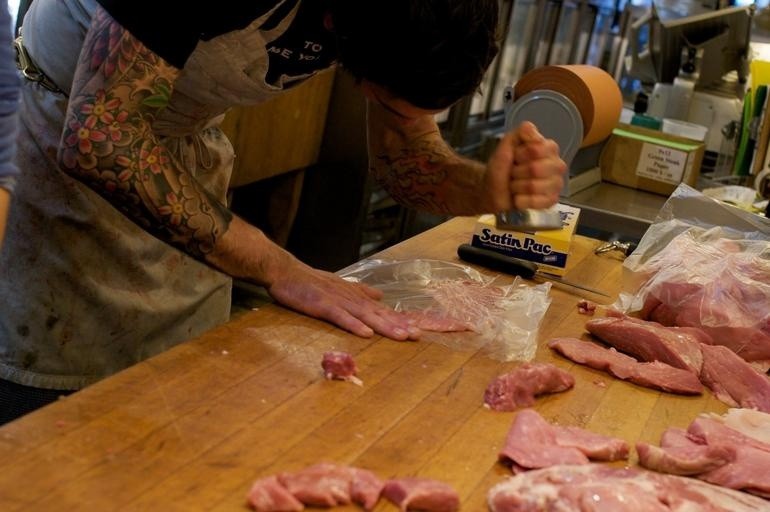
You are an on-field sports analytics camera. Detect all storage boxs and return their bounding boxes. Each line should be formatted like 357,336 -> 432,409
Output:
599,122 -> 705,195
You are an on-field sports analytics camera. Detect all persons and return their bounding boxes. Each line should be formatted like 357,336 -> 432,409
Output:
1,1 -> 568,426
1,0 -> 25,256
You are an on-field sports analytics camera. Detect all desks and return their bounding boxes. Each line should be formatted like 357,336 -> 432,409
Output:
0,216 -> 770,512
557,168 -> 668,238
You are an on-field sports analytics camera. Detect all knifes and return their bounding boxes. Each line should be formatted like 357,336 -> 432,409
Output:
453,244 -> 614,300
